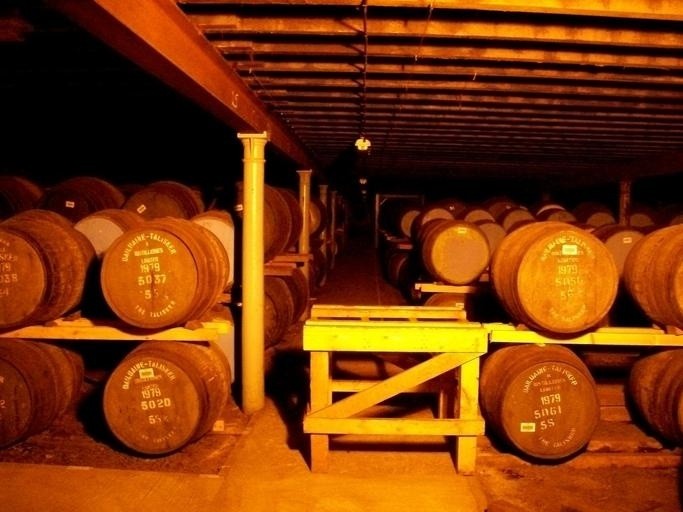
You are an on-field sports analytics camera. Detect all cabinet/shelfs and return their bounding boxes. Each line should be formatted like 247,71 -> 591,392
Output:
301,302 -> 485,474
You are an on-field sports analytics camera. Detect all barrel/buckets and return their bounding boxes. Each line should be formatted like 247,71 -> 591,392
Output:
383,197 -> 682,464
0,168 -> 344,455
